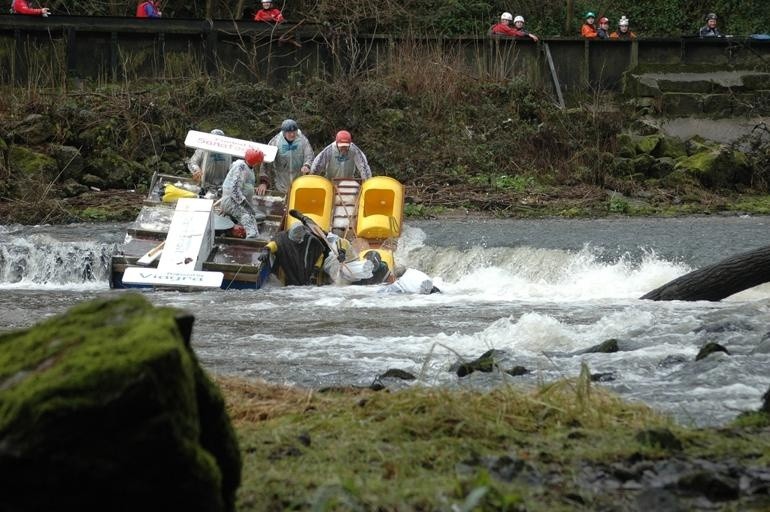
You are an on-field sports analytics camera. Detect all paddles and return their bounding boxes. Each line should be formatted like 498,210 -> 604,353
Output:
136,198 -> 222,265
307,223 -> 355,275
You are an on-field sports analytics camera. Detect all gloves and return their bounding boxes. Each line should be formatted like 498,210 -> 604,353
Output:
338,248 -> 346,262
258,247 -> 270,264
309,267 -> 319,283
242,200 -> 254,214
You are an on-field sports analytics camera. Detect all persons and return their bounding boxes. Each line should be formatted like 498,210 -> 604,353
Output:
486,11 -> 539,41
255,118 -> 314,198
289,210 -> 394,286
579,12 -> 598,39
596,16 -> 610,38
609,15 -> 637,39
135,0 -> 164,19
187,128 -> 233,182
310,130 -> 373,185
6,0 -> 53,18
220,148 -> 263,241
252,0 -> 284,22
699,12 -> 734,39
512,14 -> 527,30
258,223 -> 325,286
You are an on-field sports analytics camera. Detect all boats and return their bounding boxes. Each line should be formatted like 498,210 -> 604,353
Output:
109,129 -> 290,292
275,172 -> 404,285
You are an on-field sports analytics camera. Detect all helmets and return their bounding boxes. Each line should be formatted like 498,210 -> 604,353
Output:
706,13 -> 717,21
282,120 -> 297,131
501,12 -> 512,20
245,150 -> 263,166
514,16 -> 524,23
336,131 -> 351,147
586,12 -> 629,26
364,250 -> 381,272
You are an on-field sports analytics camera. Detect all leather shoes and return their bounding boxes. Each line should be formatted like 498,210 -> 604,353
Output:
289,210 -> 303,220
